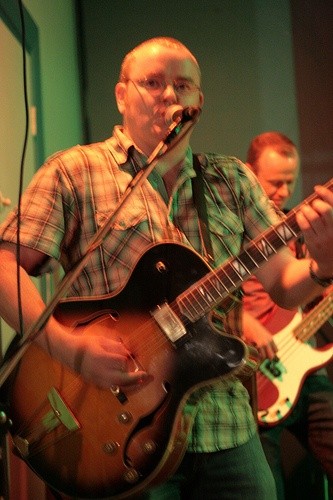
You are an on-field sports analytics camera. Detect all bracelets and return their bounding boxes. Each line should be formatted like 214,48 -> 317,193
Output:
309,258 -> 333,289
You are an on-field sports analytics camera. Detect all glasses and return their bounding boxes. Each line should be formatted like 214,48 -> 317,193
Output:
125,77 -> 202,95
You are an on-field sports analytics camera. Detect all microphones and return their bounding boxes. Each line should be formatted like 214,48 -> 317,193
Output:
165,104 -> 201,123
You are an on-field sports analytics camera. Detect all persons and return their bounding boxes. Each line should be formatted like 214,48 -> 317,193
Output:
0,36 -> 333,500
238,132 -> 333,500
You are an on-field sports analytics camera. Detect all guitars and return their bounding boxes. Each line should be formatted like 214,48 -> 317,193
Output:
0,178 -> 333,500
229,290 -> 333,425
